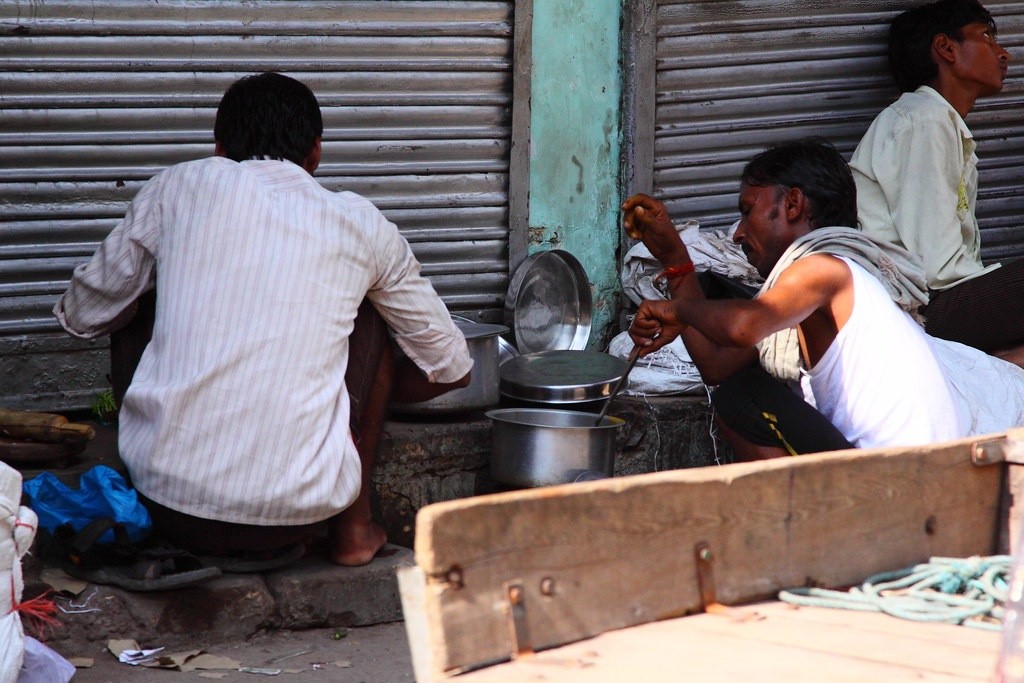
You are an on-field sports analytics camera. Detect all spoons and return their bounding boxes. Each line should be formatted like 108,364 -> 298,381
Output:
590,343 -> 643,429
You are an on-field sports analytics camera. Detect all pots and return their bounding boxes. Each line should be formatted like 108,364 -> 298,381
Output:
481,404 -> 627,491
387,320 -> 510,412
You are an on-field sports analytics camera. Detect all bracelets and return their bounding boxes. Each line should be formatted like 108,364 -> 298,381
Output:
653,261 -> 696,285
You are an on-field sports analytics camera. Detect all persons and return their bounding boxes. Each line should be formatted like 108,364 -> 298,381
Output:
619,139 -> 977,464
847,2 -> 1014,356
50,70 -> 474,568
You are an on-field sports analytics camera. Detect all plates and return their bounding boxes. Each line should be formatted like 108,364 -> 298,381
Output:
503,248 -> 594,357
497,347 -> 632,404
